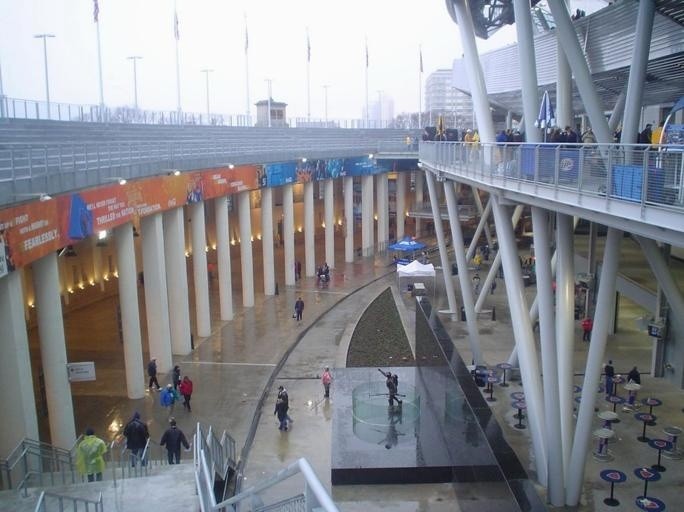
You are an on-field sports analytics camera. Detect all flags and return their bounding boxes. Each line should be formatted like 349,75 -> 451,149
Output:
365,44 -> 370,68
245,26 -> 249,54
93,0 -> 98,23
419,50 -> 424,75
174,12 -> 179,39
307,36 -> 311,64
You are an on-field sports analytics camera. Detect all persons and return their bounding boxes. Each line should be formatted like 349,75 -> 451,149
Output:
490,279 -> 497,295
207,263 -> 215,289
276,384 -> 294,428
172,365 -> 181,390
160,417 -> 190,464
316,265 -> 323,283
464,120 -> 666,165
79,427 -> 107,482
160,384 -> 180,422
295,258 -> 302,279
582,315 -> 593,342
123,412 -> 149,468
295,297 -> 304,321
472,273 -> 481,295
604,359 -> 615,394
276,220 -> 283,243
323,262 -> 331,281
374,414 -> 405,449
626,365 -> 641,404
179,375 -> 193,412
421,251 -> 428,264
148,357 -> 162,391
474,247 -> 483,269
273,393 -> 288,431
378,367 -> 406,405
322,365 -> 332,398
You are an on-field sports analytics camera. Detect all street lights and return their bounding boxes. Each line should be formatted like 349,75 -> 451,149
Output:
322,84 -> 329,129
264,79 -> 274,127
33,34 -> 56,120
377,89 -> 384,128
126,56 -> 142,124
426,93 -> 434,127
201,69 -> 212,125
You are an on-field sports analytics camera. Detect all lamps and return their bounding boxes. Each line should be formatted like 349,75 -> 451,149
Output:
167,168 -> 181,177
17,192 -> 53,202
105,177 -> 126,186
210,162 -> 234,169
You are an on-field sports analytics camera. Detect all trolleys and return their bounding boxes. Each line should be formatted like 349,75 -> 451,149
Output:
642,143 -> 675,205
513,144 -> 582,188
598,143 -> 626,196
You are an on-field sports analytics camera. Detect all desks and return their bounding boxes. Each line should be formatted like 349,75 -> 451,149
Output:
471,361 -> 527,433
570,375 -> 684,512
414,283 -> 425,297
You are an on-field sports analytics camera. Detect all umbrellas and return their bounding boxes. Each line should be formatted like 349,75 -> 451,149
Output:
437,113 -> 445,142
533,89 -> 554,143
